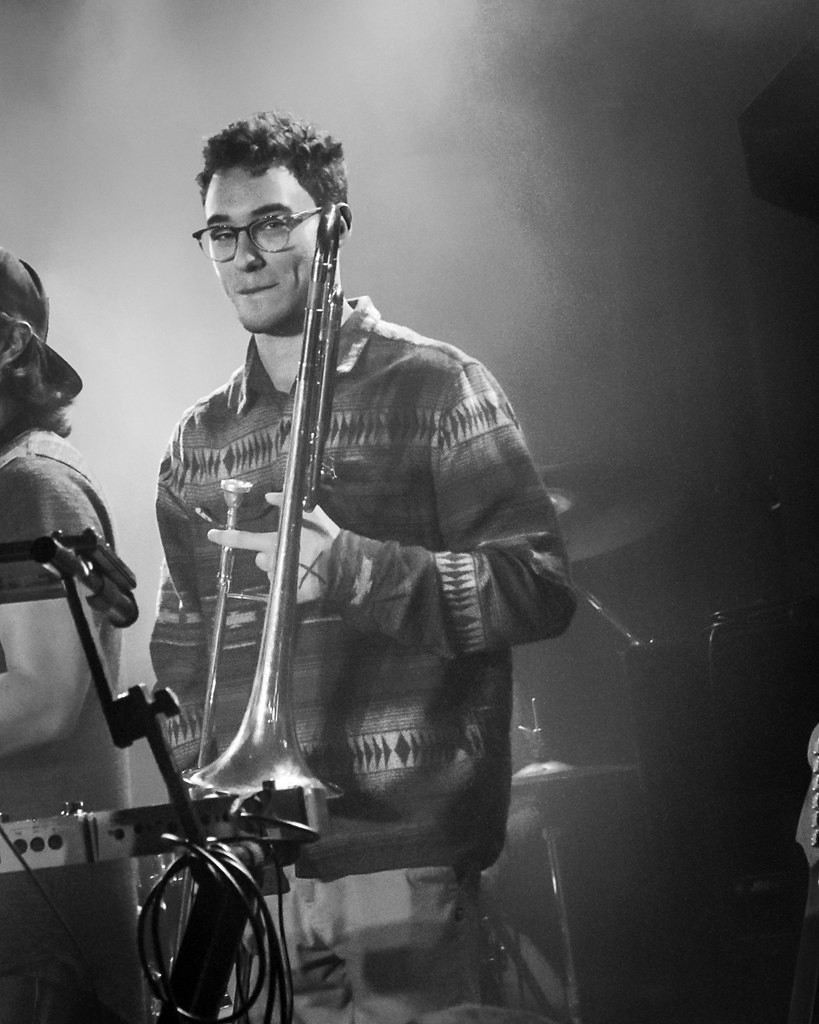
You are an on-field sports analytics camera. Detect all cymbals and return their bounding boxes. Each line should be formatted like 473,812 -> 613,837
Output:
513,757 -> 645,785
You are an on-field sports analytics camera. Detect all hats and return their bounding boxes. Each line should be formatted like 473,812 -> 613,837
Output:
0,246 -> 83,404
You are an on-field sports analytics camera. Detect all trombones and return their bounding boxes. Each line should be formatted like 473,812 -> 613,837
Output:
170,198 -> 348,979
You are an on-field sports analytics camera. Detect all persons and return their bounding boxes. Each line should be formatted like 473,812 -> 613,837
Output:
0,245 -> 152,1024
147,111 -> 579,1024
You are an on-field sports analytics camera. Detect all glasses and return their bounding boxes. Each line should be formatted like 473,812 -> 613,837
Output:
192,206 -> 323,263
